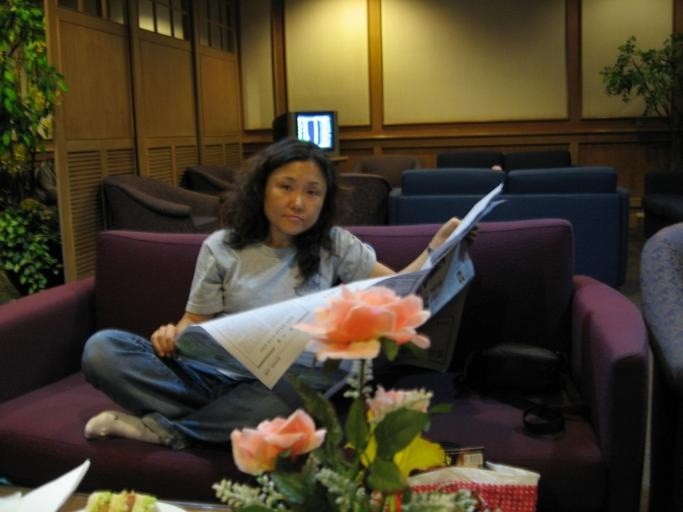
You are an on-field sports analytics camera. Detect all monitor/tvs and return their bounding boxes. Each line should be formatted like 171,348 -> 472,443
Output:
273,111 -> 340,156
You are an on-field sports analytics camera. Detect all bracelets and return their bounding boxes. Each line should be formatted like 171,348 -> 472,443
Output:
426,244 -> 433,255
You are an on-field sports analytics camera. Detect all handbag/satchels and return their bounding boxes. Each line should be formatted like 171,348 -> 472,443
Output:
383,460 -> 541,512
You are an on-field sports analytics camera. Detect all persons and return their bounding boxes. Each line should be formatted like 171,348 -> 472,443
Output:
82,139 -> 479,448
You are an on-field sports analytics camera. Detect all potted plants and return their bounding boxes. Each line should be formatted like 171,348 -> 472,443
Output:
1,0 -> 66,298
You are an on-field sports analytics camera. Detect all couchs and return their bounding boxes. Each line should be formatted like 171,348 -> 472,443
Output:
641,224 -> 682,380
98,147 -> 631,287
641,168 -> 682,236
2,220 -> 655,512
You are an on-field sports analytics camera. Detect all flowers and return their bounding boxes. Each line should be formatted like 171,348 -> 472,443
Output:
84,282 -> 495,512
